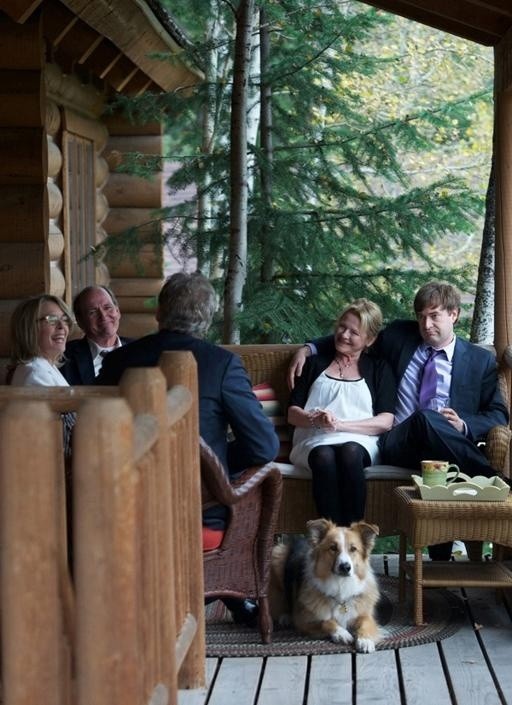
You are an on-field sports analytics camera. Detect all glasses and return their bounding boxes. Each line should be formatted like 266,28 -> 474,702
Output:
37,315 -> 69,325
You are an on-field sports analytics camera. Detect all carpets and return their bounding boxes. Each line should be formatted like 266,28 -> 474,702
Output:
199,542 -> 466,655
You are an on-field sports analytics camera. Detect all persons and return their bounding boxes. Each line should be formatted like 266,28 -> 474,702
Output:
6,293 -> 81,524
286,281 -> 512,561
92,271 -> 282,627
285,297 -> 398,535
60,285 -> 139,385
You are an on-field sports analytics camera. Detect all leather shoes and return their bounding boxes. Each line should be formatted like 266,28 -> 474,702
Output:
224,598 -> 259,626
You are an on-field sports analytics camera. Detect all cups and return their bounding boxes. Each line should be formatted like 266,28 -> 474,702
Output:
420,459 -> 460,488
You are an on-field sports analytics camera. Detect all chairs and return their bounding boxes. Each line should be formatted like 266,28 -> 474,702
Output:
186,430 -> 283,640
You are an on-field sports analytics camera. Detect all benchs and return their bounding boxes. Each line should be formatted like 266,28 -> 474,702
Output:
176,345 -> 510,539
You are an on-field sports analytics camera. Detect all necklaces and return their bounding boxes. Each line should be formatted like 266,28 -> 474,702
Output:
329,355 -> 362,378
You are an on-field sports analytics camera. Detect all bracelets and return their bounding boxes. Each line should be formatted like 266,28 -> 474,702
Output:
309,417 -> 316,428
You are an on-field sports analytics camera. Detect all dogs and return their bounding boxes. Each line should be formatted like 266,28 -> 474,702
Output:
269,516 -> 392,653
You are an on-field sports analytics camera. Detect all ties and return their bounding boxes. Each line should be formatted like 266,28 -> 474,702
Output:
419,349 -> 441,410
